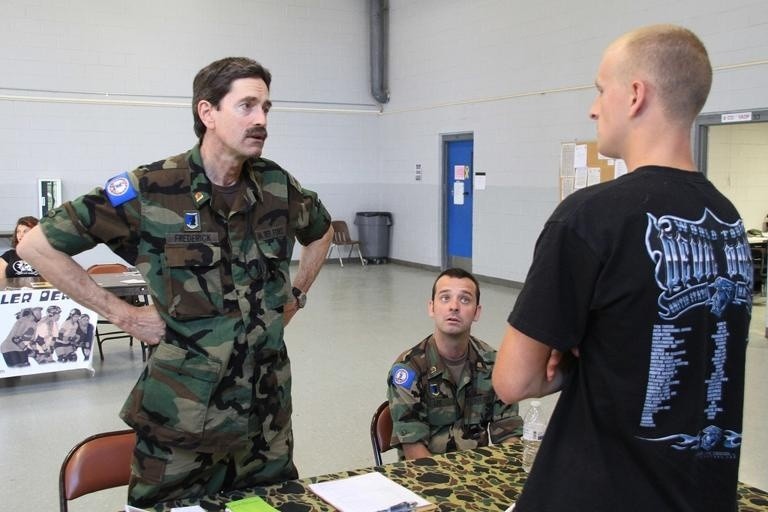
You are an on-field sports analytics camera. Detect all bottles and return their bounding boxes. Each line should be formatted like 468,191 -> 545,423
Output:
522,400 -> 548,473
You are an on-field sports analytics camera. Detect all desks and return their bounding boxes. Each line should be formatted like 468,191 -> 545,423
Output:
0,265 -> 150,379
137,441 -> 542,512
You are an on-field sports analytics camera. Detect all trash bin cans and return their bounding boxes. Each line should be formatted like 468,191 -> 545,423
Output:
354,212 -> 392,264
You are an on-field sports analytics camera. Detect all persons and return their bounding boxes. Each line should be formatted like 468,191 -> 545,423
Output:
0,216 -> 46,277
492,24 -> 753,509
386,267 -> 523,459
0,305 -> 94,369
16,57 -> 336,506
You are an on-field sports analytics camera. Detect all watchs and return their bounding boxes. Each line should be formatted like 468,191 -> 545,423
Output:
289,285 -> 306,309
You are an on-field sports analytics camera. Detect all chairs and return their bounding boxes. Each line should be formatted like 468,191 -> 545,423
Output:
326,219 -> 368,268
365,400 -> 406,467
83,262 -> 149,362
54,429 -> 142,512
